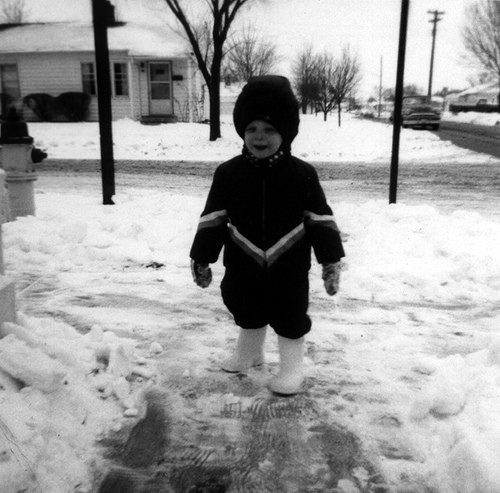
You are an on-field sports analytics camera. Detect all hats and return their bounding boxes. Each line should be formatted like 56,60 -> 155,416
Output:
233,76 -> 300,148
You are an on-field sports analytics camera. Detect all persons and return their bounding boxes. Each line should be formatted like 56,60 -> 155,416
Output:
190,74 -> 347,395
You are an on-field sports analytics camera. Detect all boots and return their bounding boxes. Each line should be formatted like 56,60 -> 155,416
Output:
220,322 -> 268,376
267,334 -> 306,394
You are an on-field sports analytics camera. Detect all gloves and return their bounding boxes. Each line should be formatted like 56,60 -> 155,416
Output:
190,258 -> 213,288
321,261 -> 341,295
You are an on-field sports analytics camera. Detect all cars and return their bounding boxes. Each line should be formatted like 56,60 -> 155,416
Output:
389,95 -> 441,130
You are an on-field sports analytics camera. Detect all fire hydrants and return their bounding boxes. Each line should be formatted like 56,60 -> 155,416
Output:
0,107 -> 48,220
